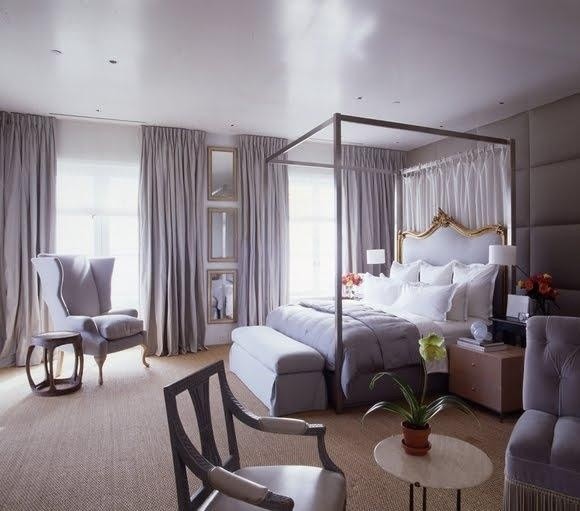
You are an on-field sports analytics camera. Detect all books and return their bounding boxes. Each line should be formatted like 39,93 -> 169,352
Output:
457,336 -> 508,353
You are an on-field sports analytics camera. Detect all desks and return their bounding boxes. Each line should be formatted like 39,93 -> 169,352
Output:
25,331 -> 84,396
374,433 -> 493,510
489,315 -> 527,349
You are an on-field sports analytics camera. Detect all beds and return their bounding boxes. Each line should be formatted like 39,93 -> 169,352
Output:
264,112 -> 519,417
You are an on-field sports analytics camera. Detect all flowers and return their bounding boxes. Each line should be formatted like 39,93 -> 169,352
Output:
359,331 -> 486,434
516,272 -> 561,316
341,272 -> 363,287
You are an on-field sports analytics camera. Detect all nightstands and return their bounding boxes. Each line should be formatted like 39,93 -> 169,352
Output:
446,340 -> 526,422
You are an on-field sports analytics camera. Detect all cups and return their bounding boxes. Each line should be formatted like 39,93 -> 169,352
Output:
517,313 -> 529,324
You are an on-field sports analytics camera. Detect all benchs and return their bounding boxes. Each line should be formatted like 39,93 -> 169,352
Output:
227,323 -> 329,418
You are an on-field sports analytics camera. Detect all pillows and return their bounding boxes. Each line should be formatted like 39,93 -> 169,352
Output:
450,260 -> 499,326
416,259 -> 453,287
388,260 -> 419,284
363,271 -> 469,324
91,314 -> 144,340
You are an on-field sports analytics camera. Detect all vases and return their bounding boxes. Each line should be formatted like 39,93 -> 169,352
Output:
527,298 -> 551,317
399,418 -> 432,457
344,285 -> 357,298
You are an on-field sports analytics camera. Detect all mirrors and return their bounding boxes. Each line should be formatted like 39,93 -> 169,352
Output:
206,270 -> 238,325
207,147 -> 238,201
206,207 -> 238,263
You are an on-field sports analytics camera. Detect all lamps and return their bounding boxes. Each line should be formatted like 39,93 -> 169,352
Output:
366,247 -> 386,276
487,244 -> 520,319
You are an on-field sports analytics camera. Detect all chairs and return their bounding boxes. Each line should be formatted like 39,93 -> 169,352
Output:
163,361 -> 346,510
501,315 -> 580,510
30,253 -> 150,386
